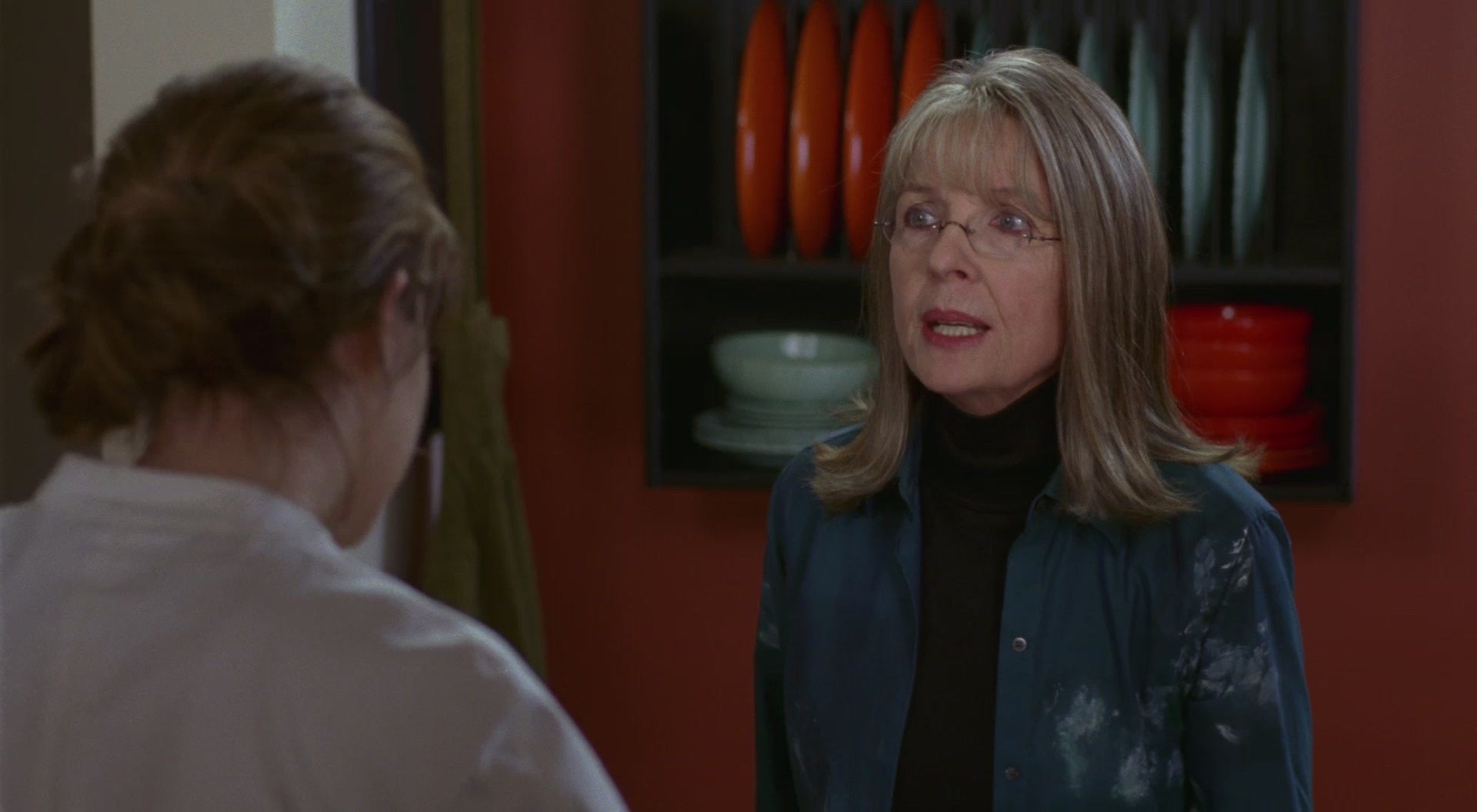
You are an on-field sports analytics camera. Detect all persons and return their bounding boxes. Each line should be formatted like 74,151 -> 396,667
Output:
0,54 -> 632,812
752,44 -> 1316,811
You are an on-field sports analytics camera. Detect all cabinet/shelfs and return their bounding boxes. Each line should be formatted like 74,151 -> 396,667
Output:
636,0 -> 1358,514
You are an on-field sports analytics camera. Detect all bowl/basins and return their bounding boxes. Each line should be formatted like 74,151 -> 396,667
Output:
1163,303 -> 1312,414
708,331 -> 879,413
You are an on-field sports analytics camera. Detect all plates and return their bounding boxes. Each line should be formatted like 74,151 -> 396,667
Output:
1230,0 -> 1272,269
1182,0 -> 1214,263
842,0 -> 895,261
897,0 -> 945,123
1184,399 -> 1333,475
788,0 -> 842,261
1126,0 -> 1161,189
965,0 -> 1107,101
734,0 -> 788,262
686,407 -> 870,471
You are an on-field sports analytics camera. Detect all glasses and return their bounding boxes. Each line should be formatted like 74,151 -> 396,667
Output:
873,190 -> 1064,256
